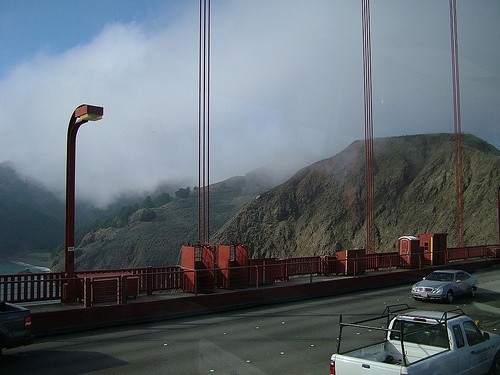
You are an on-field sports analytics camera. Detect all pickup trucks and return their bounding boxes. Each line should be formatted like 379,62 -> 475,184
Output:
330,309 -> 500,375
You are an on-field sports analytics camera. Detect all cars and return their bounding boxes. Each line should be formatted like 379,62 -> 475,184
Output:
411,269 -> 479,303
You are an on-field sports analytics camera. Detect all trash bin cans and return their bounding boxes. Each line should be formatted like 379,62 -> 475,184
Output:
336,249 -> 367,275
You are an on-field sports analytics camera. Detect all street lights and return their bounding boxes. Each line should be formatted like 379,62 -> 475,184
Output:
62,104 -> 105,304
